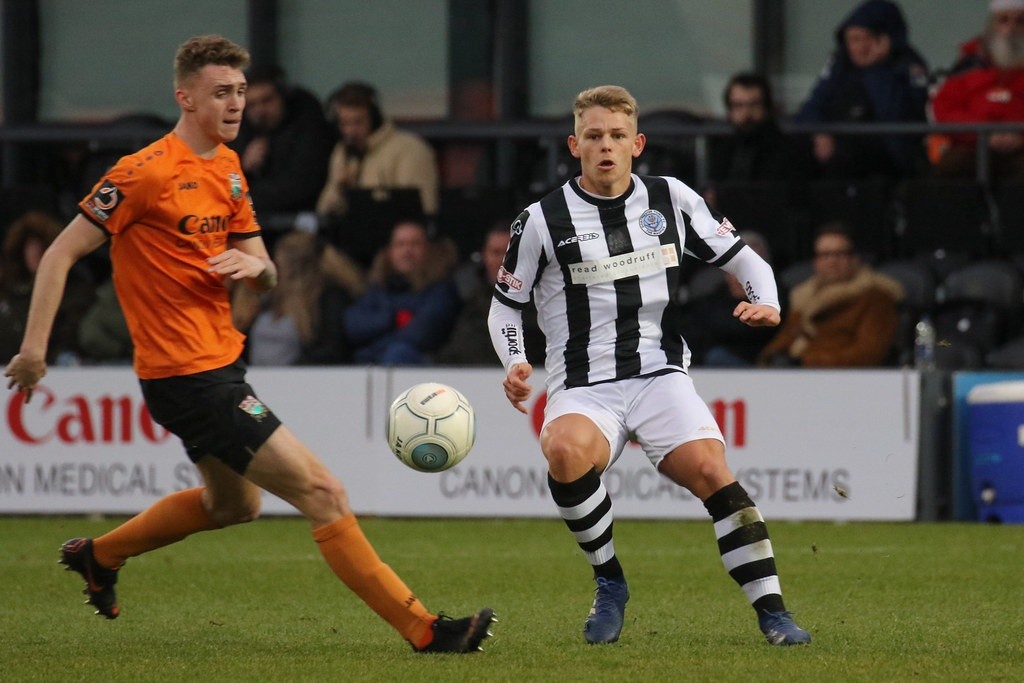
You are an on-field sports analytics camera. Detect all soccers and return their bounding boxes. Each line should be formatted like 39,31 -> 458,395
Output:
384,382 -> 477,473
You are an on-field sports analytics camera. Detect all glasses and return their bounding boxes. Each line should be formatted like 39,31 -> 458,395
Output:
728,100 -> 764,111
819,250 -> 849,262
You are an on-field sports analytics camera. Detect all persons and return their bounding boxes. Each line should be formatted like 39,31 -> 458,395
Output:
0,0 -> 1024,370
488,85 -> 811,646
4,35 -> 498,654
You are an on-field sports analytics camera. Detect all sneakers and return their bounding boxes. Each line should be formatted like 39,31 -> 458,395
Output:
583,577 -> 630,645
759,609 -> 811,645
57,538 -> 127,619
407,607 -> 498,653
